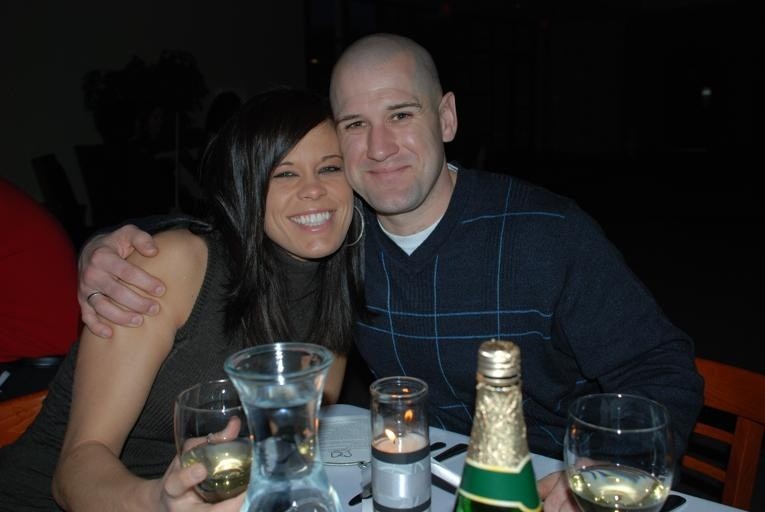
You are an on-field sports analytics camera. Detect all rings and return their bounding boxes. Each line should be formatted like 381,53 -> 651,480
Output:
87,291 -> 100,304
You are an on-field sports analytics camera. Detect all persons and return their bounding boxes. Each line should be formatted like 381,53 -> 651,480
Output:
0,89 -> 366,512
73,31 -> 706,512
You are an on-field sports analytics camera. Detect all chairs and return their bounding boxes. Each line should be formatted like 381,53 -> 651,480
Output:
680,356 -> 765,512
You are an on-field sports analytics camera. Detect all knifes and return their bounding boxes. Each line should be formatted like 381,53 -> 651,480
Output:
349,438 -> 469,507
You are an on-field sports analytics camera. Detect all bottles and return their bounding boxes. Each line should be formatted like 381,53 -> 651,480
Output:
367,376 -> 433,510
223,341 -> 346,511
450,338 -> 544,512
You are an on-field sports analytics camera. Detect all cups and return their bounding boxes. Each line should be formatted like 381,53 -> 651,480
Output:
172,377 -> 248,505
562,390 -> 679,512
